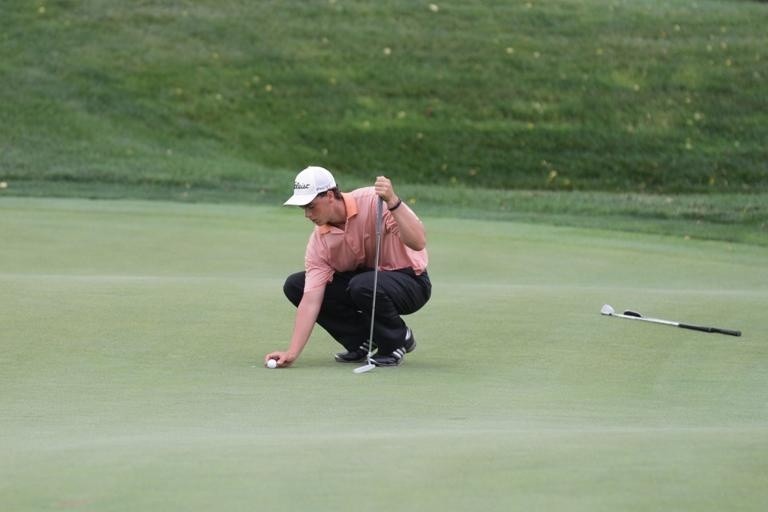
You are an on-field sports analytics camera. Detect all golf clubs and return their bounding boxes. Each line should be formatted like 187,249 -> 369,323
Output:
353,194 -> 383,374
600,304 -> 742,336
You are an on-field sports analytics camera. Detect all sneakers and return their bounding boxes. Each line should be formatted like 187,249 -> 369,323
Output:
334,337 -> 379,363
367,327 -> 417,369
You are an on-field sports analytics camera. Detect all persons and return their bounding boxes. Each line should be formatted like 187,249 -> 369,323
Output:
262,164 -> 434,374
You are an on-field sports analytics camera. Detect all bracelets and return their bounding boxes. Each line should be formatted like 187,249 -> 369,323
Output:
386,199 -> 403,212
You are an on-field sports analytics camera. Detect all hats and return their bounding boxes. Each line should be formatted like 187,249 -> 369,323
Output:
283,166 -> 336,206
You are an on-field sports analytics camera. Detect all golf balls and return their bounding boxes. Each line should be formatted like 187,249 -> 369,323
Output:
267,359 -> 277,369
